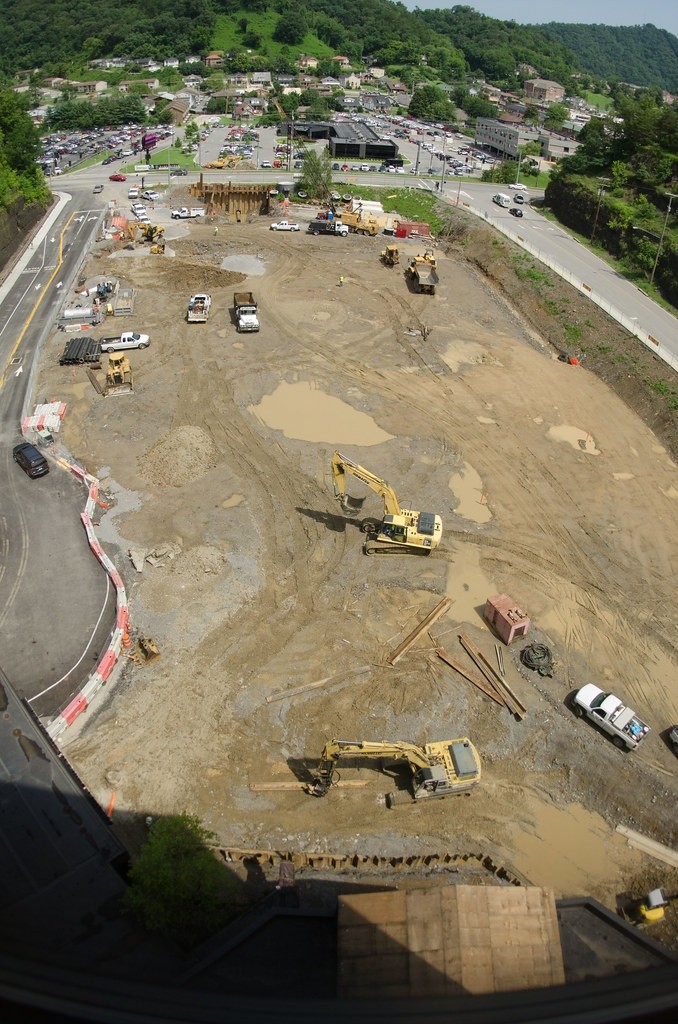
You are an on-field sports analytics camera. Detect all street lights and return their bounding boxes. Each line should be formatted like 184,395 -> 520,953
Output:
588,176 -> 610,244
648,193 -> 678,288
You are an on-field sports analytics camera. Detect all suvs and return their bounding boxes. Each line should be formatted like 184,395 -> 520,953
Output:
13,443 -> 49,479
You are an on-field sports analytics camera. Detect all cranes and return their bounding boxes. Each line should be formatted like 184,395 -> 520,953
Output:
273,97 -> 381,237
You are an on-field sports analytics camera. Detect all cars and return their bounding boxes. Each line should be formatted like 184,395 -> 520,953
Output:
94,184 -> 103,193
136,214 -> 151,226
509,183 -> 528,191
131,203 -> 147,217
327,109 -> 499,178
509,208 -> 523,217
514,194 -> 524,204
35,97 -> 308,175
128,186 -> 139,199
140,190 -> 159,201
109,174 -> 127,182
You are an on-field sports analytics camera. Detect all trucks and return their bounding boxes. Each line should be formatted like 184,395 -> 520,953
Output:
407,256 -> 439,295
171,207 -> 205,220
309,219 -> 349,237
234,292 -> 260,333
188,293 -> 212,323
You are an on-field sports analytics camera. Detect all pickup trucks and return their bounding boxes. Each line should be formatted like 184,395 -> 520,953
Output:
270,220 -> 299,232
100,332 -> 151,353
570,682 -> 653,753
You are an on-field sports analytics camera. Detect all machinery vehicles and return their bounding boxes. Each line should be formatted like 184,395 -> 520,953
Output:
418,249 -> 438,270
125,223 -> 165,252
619,887 -> 671,931
103,352 -> 134,398
381,245 -> 400,265
329,449 -> 444,559
303,737 -> 483,812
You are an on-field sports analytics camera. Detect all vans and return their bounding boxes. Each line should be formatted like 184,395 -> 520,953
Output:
492,193 -> 511,208
